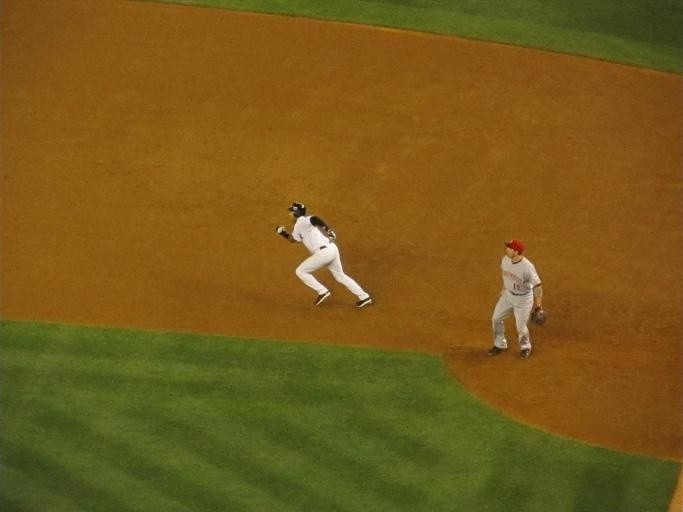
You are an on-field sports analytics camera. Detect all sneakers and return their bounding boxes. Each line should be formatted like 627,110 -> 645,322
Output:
488,344 -> 506,355
355,296 -> 372,307
314,291 -> 332,305
520,346 -> 533,358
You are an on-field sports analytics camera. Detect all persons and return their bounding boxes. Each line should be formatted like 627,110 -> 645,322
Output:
488,239 -> 546,361
275,202 -> 372,309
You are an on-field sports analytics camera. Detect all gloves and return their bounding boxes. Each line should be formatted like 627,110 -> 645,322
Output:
327,227 -> 336,240
274,225 -> 289,237
531,306 -> 546,326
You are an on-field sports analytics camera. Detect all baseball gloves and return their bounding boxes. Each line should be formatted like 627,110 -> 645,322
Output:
531,305 -> 548,327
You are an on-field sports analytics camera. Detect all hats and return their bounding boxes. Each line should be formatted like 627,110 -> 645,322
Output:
505,239 -> 524,254
289,203 -> 306,214
